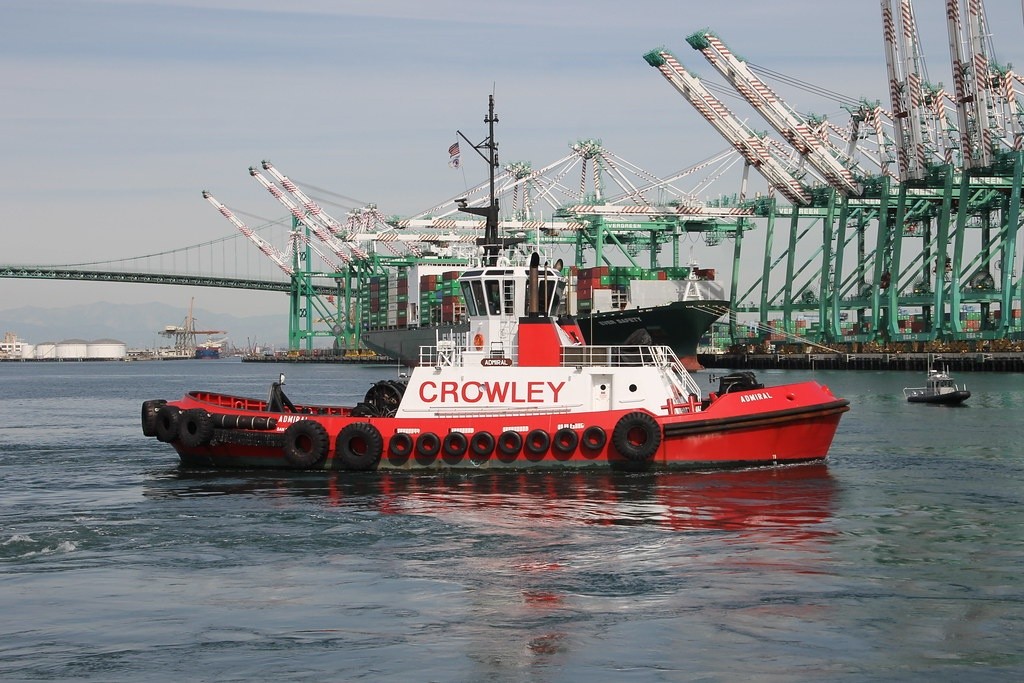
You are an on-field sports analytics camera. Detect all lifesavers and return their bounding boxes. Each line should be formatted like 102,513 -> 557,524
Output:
612,411 -> 662,461
389,432 -> 413,457
138,398 -> 214,450
498,430 -> 522,456
471,431 -> 495,456
334,421 -> 384,471
416,431 -> 441,456
582,426 -> 606,450
279,420 -> 331,471
554,428 -> 578,453
444,431 -> 468,457
473,334 -> 483,350
525,428 -> 551,454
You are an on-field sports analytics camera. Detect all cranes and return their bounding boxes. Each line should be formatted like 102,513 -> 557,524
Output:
158,297 -> 227,346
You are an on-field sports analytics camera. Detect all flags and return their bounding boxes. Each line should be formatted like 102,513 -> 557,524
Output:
448,142 -> 460,158
448,153 -> 464,169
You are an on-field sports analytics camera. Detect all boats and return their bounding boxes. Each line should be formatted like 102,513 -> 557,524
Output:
139,94 -> 852,476
901,352 -> 972,404
